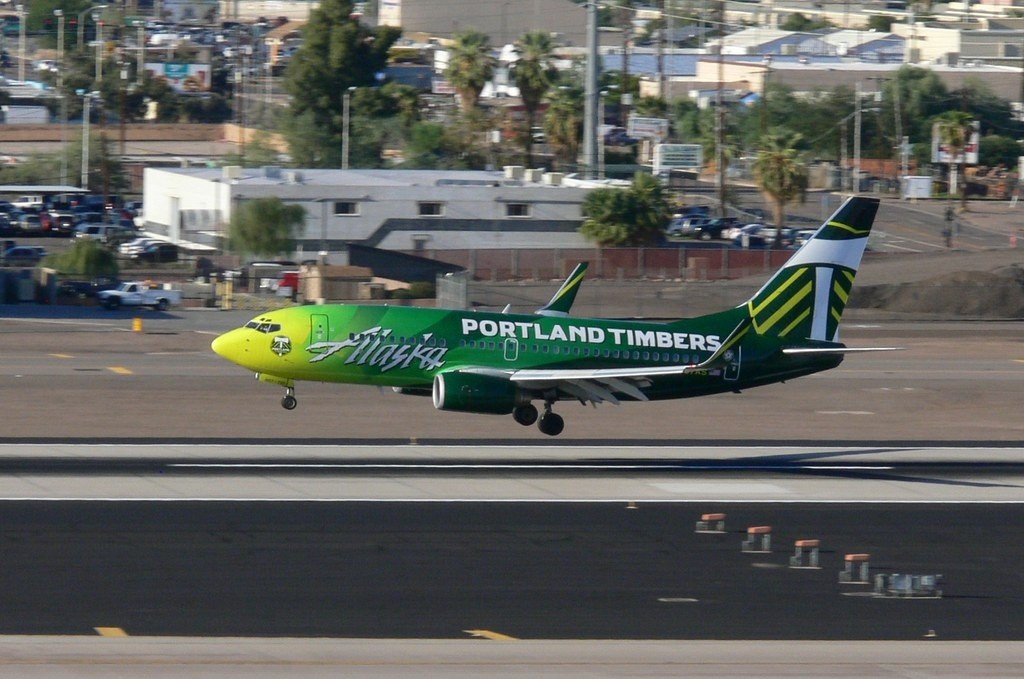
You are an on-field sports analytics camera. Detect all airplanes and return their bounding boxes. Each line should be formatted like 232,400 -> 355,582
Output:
211,194 -> 904,437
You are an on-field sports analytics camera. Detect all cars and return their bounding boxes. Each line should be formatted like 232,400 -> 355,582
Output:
0,191 -> 179,270
132,20 -> 303,83
418,93 -> 817,247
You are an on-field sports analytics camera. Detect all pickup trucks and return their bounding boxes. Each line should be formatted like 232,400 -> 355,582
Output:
100,281 -> 181,310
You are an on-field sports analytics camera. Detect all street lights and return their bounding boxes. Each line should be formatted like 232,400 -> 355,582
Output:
53,10 -> 64,87
77,90 -> 101,191
81,5 -> 107,57
138,27 -> 144,85
16,4 -> 25,81
